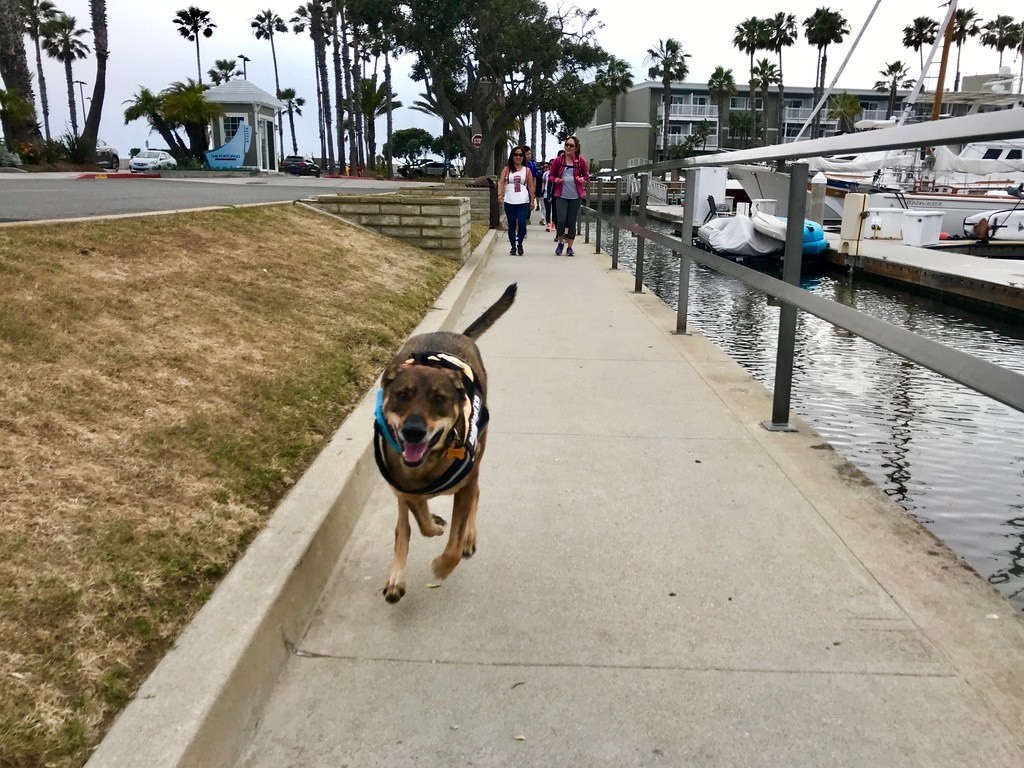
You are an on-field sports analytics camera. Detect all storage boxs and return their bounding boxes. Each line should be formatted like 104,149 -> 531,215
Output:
751,198 -> 778,216
903,210 -> 946,246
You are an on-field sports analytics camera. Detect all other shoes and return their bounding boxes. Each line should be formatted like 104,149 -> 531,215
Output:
516,234 -> 519,240
539,219 -> 545,225
524,230 -> 528,239
563,234 -> 568,239
527,220 -> 531,225
535,207 -> 540,210
554,236 -> 558,241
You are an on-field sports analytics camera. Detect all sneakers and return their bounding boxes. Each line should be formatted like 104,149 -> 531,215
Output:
545,224 -> 551,232
552,224 -> 555,230
567,247 -> 574,256
510,247 -> 517,254
517,244 -> 523,255
556,243 -> 564,255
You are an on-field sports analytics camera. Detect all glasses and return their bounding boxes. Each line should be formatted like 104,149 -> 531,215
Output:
513,153 -> 523,157
543,166 -> 548,168
565,143 -> 575,147
525,153 -> 531,155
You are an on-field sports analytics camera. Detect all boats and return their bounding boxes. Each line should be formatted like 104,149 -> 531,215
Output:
697,209 -> 778,260
720,0 -> 1024,243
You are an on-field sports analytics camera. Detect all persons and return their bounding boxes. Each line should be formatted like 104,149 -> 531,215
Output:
497,136 -> 589,256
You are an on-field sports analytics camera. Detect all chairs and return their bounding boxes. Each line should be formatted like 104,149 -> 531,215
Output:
703,195 -> 729,224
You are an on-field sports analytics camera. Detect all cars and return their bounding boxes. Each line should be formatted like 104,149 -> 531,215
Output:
128,150 -> 177,173
67,138 -> 120,172
406,161 -> 457,179
327,161 -> 346,172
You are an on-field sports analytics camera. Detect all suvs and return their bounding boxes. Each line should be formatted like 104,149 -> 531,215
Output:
282,155 -> 321,179
397,158 -> 434,178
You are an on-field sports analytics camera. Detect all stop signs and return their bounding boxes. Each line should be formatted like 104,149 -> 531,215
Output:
472,133 -> 483,148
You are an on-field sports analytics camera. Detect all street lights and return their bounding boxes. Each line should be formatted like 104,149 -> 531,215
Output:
75,81 -> 87,126
237,54 -> 251,80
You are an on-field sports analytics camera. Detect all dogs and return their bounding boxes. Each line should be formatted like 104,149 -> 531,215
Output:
374,282 -> 518,605
